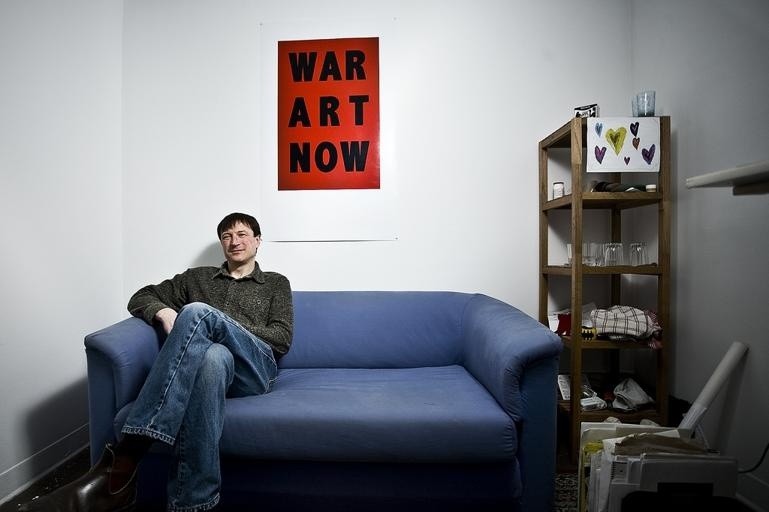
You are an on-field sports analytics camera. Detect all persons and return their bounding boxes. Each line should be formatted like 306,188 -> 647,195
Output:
12,212 -> 293,512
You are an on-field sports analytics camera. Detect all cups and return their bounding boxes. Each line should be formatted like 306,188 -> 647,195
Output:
567,241 -> 625,266
631,90 -> 657,118
628,241 -> 649,266
552,181 -> 565,199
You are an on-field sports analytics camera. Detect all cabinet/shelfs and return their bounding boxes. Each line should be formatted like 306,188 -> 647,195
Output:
538,114 -> 672,474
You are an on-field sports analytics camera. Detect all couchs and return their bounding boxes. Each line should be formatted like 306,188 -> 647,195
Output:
81,290 -> 558,510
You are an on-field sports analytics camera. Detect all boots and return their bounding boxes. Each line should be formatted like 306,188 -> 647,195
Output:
16,443 -> 144,512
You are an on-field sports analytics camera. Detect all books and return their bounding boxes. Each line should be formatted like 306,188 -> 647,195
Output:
577,421 -> 740,512
